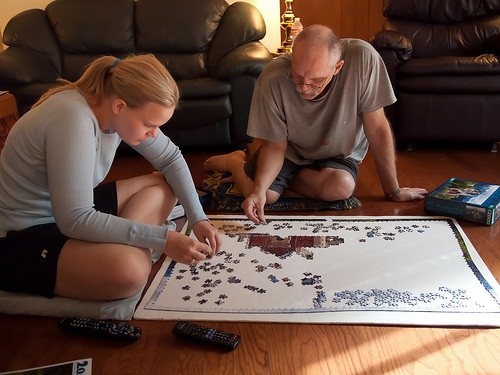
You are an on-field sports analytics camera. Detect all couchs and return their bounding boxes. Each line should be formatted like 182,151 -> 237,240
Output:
370,0 -> 500,152
0,0 -> 273,158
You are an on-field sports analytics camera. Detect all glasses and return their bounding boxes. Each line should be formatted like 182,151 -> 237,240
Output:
287,66 -> 328,90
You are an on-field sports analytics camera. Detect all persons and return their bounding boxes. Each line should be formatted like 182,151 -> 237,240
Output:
0,53 -> 222,301
204,24 -> 427,225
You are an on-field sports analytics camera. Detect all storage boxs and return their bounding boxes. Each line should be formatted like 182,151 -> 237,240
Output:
424,177 -> 500,226
